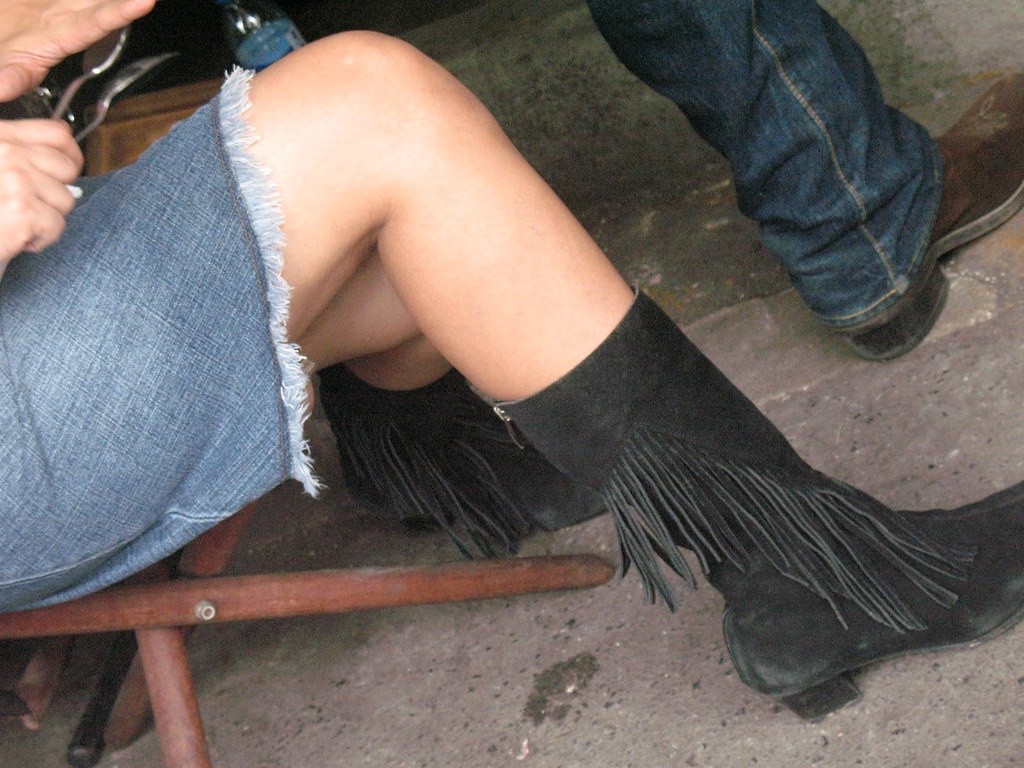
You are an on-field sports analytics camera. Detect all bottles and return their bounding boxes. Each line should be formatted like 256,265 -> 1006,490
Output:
214,0 -> 306,73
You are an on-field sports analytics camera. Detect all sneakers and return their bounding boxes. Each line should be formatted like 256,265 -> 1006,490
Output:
836,75 -> 1024,358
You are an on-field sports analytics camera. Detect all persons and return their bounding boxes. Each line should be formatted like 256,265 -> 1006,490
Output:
0,0 -> 1024,720
584,0 -> 1024,363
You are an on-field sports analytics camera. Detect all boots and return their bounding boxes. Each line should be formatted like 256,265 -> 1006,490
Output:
316,363 -> 608,562
469,283 -> 1023,723
